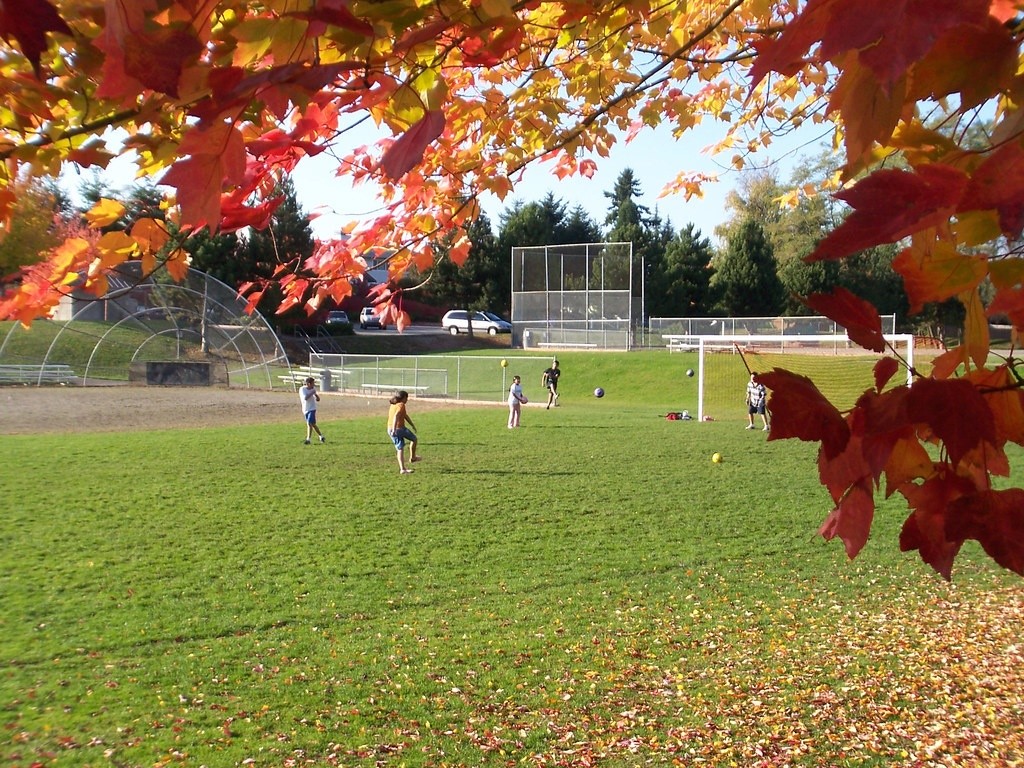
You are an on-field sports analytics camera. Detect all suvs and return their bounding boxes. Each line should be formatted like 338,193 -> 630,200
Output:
360,308 -> 387,330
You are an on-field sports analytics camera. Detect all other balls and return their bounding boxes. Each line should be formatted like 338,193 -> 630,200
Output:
521,396 -> 528,404
711,452 -> 723,464
686,368 -> 694,377
500,359 -> 508,367
594,387 -> 604,398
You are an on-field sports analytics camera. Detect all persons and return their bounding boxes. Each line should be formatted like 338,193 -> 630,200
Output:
541,360 -> 561,410
745,372 -> 769,431
298,377 -> 325,444
507,376 -> 528,428
387,391 -> 422,474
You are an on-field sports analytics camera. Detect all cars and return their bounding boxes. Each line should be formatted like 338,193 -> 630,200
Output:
325,311 -> 349,324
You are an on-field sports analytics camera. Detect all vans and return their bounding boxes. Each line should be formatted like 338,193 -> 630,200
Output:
440,310 -> 510,336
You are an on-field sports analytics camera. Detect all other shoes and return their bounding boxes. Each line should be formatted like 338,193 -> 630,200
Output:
410,456 -> 422,462
746,425 -> 755,430
303,440 -> 311,444
400,469 -> 415,474
321,437 -> 325,442
762,427 -> 769,432
